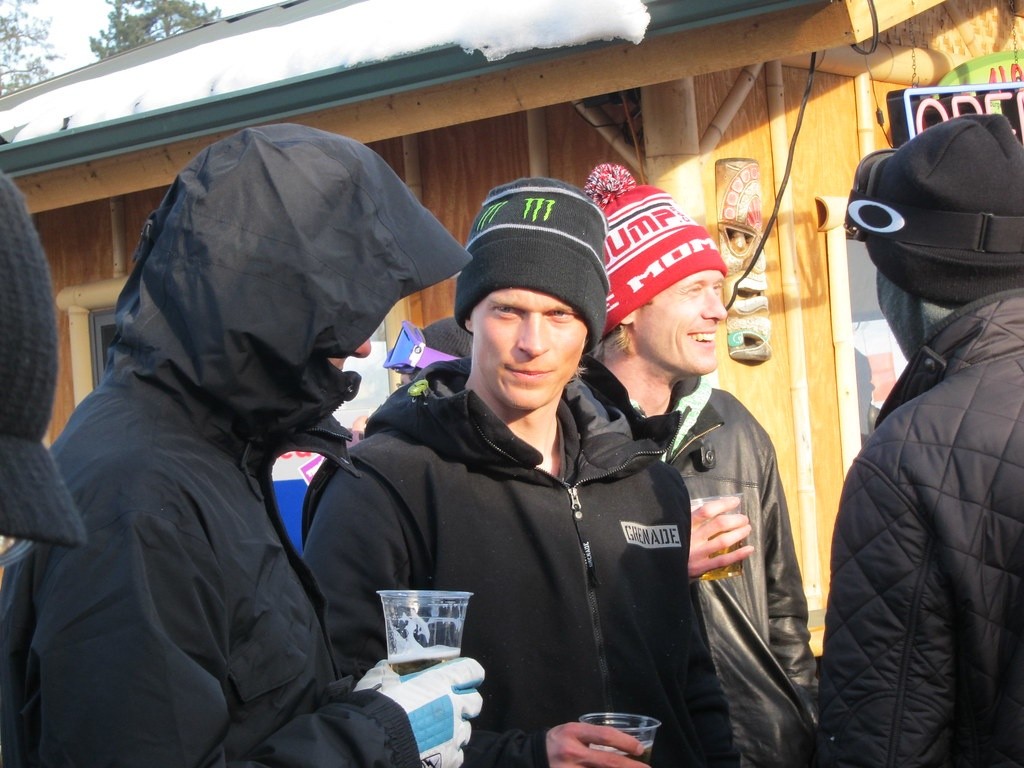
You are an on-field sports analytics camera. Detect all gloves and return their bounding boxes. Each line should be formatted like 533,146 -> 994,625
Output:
355,656 -> 484,767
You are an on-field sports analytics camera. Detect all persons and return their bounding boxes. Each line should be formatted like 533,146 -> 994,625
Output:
0,174 -> 89,550
0,122 -> 486,768
299,177 -> 740,768
812,113 -> 1024,768
584,164 -> 818,768
352,317 -> 474,432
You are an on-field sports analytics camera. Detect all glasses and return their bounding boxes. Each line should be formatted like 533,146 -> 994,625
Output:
385,320 -> 452,372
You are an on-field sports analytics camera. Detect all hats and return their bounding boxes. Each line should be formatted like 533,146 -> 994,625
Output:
864,114 -> 1024,306
418,316 -> 474,357
0,173 -> 92,547
454,178 -> 609,353
584,165 -> 728,338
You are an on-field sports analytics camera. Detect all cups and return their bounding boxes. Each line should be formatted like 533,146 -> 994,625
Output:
691,491 -> 745,580
578,713 -> 661,765
376,590 -> 474,677
